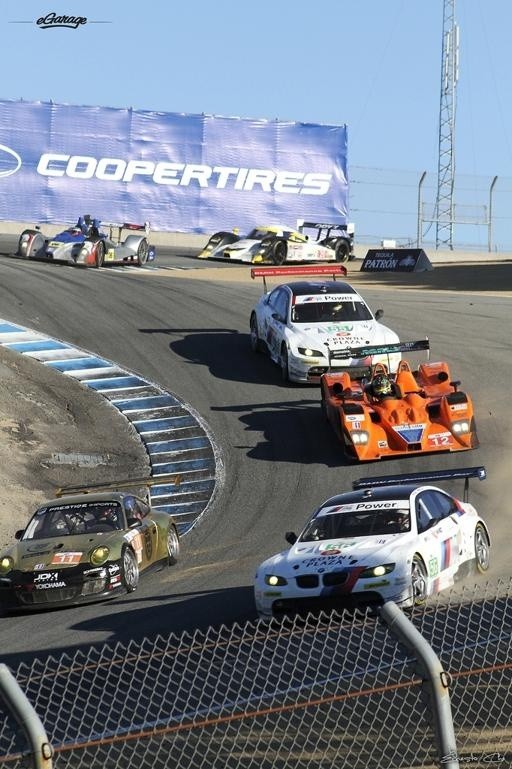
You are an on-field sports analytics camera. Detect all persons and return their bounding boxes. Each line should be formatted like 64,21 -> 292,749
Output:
70,227 -> 82,237
386,509 -> 410,531
369,374 -> 394,402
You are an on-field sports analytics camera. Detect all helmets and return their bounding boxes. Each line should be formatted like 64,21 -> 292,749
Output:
71,227 -> 81,236
328,304 -> 345,320
393,509 -> 410,525
372,375 -> 393,396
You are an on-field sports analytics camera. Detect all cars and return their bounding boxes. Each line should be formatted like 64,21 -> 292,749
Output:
319,336 -> 479,464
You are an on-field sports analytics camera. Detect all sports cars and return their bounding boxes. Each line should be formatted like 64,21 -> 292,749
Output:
254,466 -> 491,625
250,265 -> 401,387
0,474 -> 183,611
